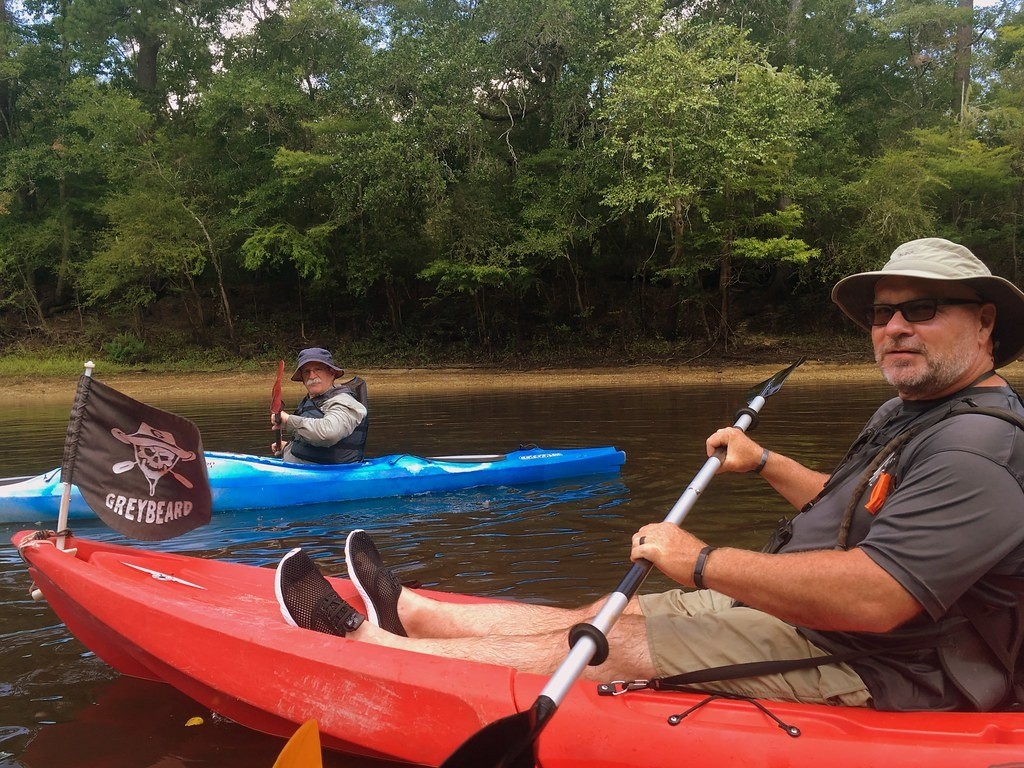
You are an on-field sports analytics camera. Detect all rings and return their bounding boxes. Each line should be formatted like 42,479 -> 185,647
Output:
640,536 -> 646,545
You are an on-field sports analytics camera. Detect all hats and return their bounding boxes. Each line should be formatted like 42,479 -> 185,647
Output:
291,347 -> 344,382
833,238 -> 1024,372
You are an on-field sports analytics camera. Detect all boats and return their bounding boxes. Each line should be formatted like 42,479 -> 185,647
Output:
13,528 -> 1024,768
0,449 -> 626,524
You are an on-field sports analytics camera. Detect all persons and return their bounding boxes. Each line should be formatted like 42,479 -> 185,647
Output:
273,240 -> 1023,712
272,348 -> 367,464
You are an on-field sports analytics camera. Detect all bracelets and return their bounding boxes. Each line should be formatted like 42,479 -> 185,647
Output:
695,546 -> 716,588
753,449 -> 769,473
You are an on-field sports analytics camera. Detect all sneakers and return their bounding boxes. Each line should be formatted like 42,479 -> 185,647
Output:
344,529 -> 408,638
274,548 -> 366,638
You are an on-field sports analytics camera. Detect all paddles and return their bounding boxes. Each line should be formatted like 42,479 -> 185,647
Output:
271,356 -> 286,458
438,350 -> 810,768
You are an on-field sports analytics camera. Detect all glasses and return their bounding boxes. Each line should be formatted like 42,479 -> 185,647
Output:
864,296 -> 983,325
303,367 -> 328,373
